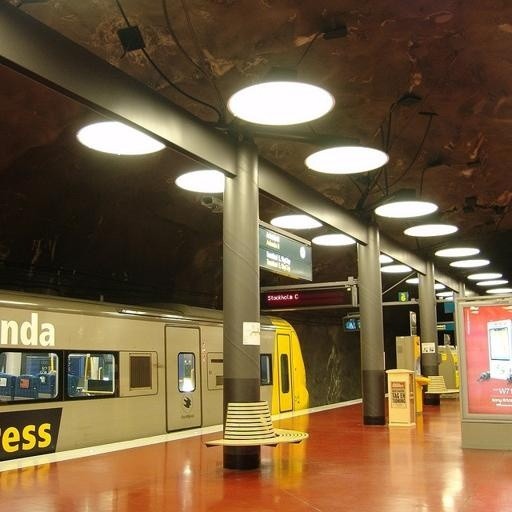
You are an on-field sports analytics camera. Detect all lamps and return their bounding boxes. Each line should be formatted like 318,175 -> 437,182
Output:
73,66 -> 512,300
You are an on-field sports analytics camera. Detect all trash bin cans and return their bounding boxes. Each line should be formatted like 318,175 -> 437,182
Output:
386,368 -> 417,427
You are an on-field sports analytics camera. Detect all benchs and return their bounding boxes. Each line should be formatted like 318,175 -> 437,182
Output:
425,375 -> 459,395
203,399 -> 309,447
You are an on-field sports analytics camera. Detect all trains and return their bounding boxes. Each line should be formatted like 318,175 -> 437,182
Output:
0,287 -> 309,464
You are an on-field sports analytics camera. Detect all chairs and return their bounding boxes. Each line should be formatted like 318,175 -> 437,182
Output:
0,371 -> 80,403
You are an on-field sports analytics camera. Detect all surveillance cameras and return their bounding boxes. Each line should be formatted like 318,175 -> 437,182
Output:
201,195 -> 223,212
